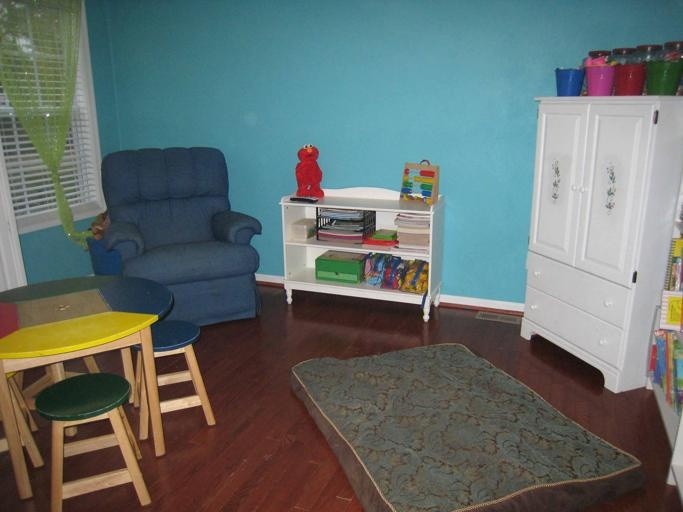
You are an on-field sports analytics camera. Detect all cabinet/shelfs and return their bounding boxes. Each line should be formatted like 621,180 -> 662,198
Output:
277,185 -> 441,323
518,95 -> 683,395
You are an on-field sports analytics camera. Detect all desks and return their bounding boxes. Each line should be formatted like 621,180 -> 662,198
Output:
1,275 -> 174,501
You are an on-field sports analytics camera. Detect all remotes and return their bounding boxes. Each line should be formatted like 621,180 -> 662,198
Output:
290,196 -> 318,203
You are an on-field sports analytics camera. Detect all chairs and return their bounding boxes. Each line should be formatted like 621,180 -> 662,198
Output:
94,146 -> 261,325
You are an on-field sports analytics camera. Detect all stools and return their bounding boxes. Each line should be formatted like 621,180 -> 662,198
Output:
130,319 -> 217,441
35,373 -> 152,512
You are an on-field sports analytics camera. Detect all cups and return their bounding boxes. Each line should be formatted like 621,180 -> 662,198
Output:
554,61 -> 683,96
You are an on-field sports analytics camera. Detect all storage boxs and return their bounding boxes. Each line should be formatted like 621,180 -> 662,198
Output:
316,249 -> 371,284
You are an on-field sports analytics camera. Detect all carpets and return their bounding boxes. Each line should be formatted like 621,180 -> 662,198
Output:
292,342 -> 646,512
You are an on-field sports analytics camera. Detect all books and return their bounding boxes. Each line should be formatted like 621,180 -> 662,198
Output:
316,208 -> 430,255
647,190 -> 683,416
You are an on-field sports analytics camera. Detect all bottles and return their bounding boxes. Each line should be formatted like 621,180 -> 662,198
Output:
583,41 -> 683,90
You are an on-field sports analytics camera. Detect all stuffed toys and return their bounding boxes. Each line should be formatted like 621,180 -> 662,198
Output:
296,144 -> 325,198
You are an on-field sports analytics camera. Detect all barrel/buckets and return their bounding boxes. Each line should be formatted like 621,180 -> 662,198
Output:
646,59 -> 683,95
554,65 -> 585,96
584,64 -> 615,95
614,63 -> 647,96
